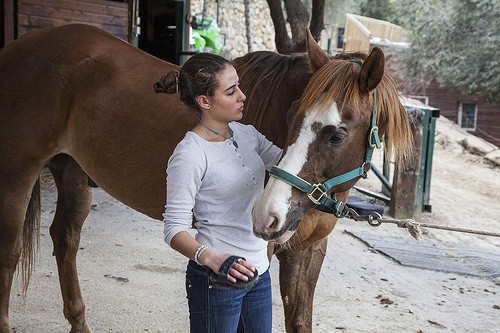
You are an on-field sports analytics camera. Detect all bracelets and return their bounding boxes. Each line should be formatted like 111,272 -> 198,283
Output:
194,245 -> 211,266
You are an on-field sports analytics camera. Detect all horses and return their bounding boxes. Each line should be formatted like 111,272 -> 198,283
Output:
0,23 -> 422,333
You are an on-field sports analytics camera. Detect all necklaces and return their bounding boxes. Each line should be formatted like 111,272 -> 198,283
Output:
198,117 -> 239,148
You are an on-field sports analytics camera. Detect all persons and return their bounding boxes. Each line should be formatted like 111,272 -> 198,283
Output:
154,52 -> 284,333
